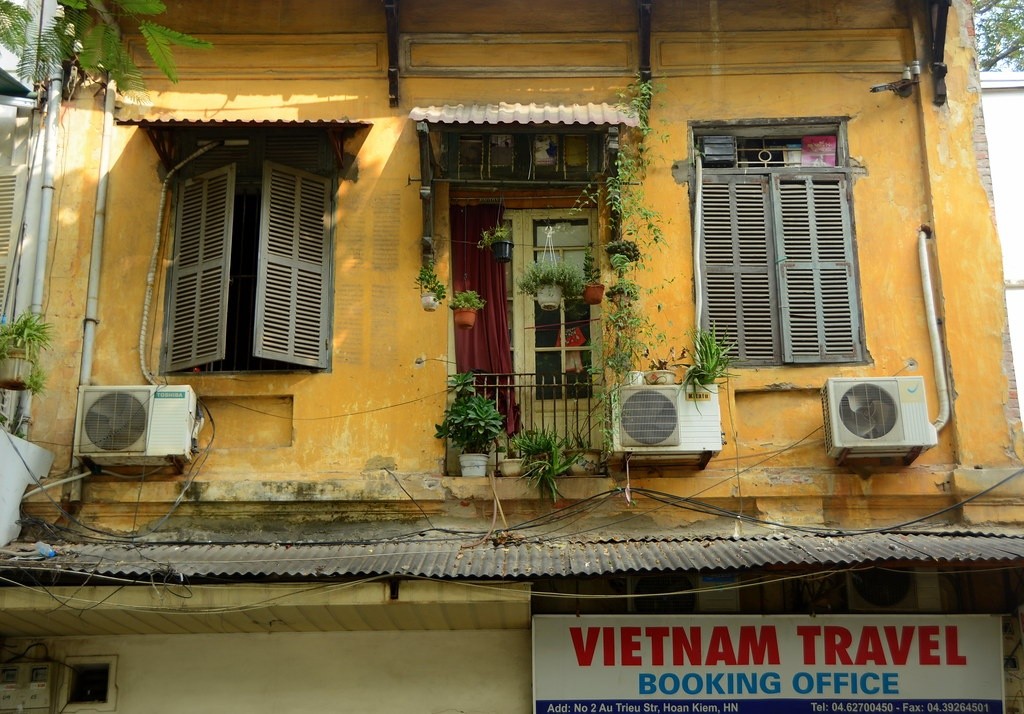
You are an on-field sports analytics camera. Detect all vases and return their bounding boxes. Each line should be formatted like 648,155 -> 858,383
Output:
624,370 -> 646,386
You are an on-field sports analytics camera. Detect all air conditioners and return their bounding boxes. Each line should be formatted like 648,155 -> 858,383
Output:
75,384 -> 200,468
821,375 -> 938,460
604,381 -> 722,463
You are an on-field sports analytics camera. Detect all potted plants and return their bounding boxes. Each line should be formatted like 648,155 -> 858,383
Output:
432,370 -> 507,477
413,240 -> 447,313
512,226 -> 586,311
579,241 -> 606,305
641,345 -> 690,386
476,196 -> 515,263
491,437 -> 527,478
562,428 -> 601,476
449,279 -> 487,330
